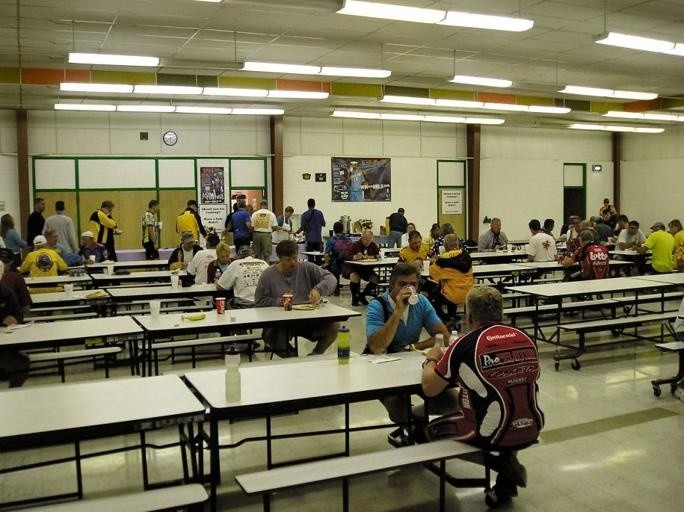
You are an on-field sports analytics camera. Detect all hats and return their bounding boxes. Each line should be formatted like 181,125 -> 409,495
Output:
207,233 -> 221,246
81,230 -> 94,237
179,230 -> 195,240
187,199 -> 200,210
237,245 -> 256,256
32,235 -> 48,245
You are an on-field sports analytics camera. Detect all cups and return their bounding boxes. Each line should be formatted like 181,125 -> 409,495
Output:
507,245 -> 511,253
90,255 -> 94,261
216,298 -> 226,313
159,222 -> 162,228
64,285 -> 74,296
149,302 -> 161,319
283,295 -> 293,311
423,261 -> 430,272
171,276 -> 179,290
399,285 -> 418,305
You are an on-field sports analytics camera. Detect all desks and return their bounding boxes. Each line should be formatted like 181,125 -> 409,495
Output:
344,258 -> 400,300
0,373 -> 205,511
468,250 -> 532,265
108,281 -> 234,315
250,241 -> 308,266
133,301 -> 363,377
300,250 -> 332,270
0,313 -> 144,391
636,271 -> 683,342
28,289 -> 111,317
86,258 -> 168,273
0,262 -> 85,276
505,234 -> 569,250
182,341 -> 462,485
608,248 -> 655,276
91,269 -> 192,283
504,277 -> 673,346
324,233 -> 395,251
380,245 -> 404,258
471,258 -> 634,292
0,272 -> 92,288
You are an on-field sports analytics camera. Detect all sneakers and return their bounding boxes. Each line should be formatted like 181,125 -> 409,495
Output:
506,452 -> 528,490
351,297 -> 359,306
359,292 -> 369,306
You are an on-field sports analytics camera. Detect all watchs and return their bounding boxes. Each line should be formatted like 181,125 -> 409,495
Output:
422,357 -> 438,367
404,344 -> 409,350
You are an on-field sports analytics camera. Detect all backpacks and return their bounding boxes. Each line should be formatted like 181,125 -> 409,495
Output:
333,234 -> 351,260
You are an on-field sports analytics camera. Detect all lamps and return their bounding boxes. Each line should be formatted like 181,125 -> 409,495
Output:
377,95 -> 571,115
600,110 -> 684,121
329,111 -> 505,126
555,85 -> 659,100
336,0 -> 534,33
58,83 -> 329,99
68,53 -> 161,66
235,62 -> 392,78
565,123 -> 665,134
447,75 -> 513,88
54,104 -> 285,116
591,32 -> 684,57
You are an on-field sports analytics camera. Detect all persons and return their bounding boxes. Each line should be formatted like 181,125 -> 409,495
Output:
26,198 -> 46,249
401,223 -> 417,248
208,243 -> 238,283
599,198 -> 617,217
223,198 -> 252,253
517,218 -> 559,281
348,162 -> 365,202
431,234 -> 475,324
186,234 -> 221,285
421,285 -> 544,506
614,214 -> 629,235
254,240 -> 339,357
168,232 -> 205,287
668,220 -> 684,272
364,265 -> 451,446
225,203 -> 239,232
87,201 -> 122,262
0,261 -> 31,388
251,200 -> 281,264
1,214 -> 29,270
45,231 -> 65,253
40,200 -> 77,256
346,231 -> 379,306
400,230 -> 431,264
387,207 -> 406,247
424,223 -> 441,245
563,232 -> 610,278
272,207 -> 294,241
142,200 -> 160,259
0,248 -> 33,316
613,220 -> 646,275
633,222 -> 675,274
561,214 -> 600,232
601,208 -> 616,227
217,245 -> 270,350
295,198 -> 326,266
426,223 -> 469,256
78,231 -> 109,262
176,200 -> 213,247
478,217 -> 513,293
542,219 -> 554,238
674,298 -> 684,407
20,236 -> 69,294
326,221 -> 352,295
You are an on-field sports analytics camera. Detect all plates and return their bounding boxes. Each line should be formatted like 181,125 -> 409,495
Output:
424,347 -> 449,353
291,304 -> 317,310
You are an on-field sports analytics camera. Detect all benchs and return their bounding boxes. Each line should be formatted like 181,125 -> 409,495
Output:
371,267 -> 393,281
0,484 -> 209,512
501,292 -> 538,329
23,312 -> 99,323
28,304 -> 91,312
469,274 -> 514,293
116,298 -> 198,315
99,282 -> 173,288
377,277 -> 389,293
138,333 -> 299,375
117,304 -> 213,317
234,437 -> 539,512
652,342 -> 684,397
502,299 -> 617,337
556,310 -> 678,371
25,346 -> 122,384
612,291 -> 684,334
25,285 -> 82,293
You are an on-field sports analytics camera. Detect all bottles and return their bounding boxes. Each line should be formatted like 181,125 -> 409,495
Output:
215,269 -> 221,280
337,326 -> 350,364
435,334 -> 445,351
224,346 -> 241,403
449,330 -> 459,345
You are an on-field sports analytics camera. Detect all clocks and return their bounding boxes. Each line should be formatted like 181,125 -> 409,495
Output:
163,131 -> 177,146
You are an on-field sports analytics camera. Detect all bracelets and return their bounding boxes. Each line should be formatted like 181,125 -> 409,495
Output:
410,344 -> 416,351
